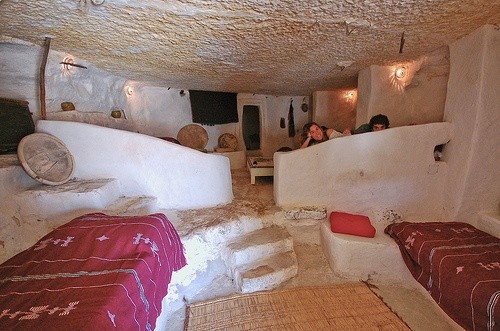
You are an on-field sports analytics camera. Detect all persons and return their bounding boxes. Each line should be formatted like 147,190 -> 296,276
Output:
350,114 -> 389,134
299,122 -> 351,149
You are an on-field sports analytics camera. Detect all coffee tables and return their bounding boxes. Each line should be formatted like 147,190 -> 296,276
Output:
247,156 -> 274,185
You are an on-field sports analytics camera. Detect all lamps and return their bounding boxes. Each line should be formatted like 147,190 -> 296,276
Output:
347,90 -> 357,99
63,57 -> 74,70
127,88 -> 133,96
396,66 -> 406,79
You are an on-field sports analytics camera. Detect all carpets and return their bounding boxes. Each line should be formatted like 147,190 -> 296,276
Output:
183,280 -> 413,331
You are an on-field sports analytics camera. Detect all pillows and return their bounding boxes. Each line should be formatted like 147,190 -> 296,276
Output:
329,211 -> 377,239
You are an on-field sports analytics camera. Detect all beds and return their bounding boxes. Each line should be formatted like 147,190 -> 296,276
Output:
383,220 -> 500,331
0,211 -> 187,331
